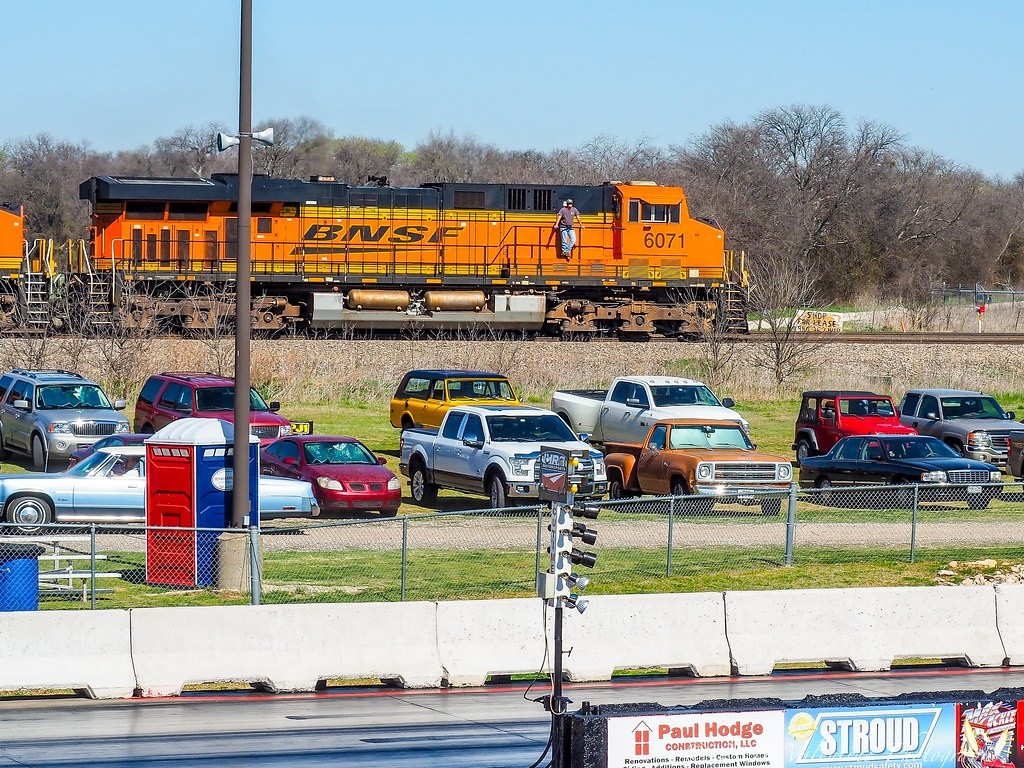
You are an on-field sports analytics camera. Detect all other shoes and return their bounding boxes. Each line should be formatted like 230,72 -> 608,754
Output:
561,251 -> 565,255
565,252 -> 570,256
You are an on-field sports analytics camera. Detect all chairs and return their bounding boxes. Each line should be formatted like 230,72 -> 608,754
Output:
674,388 -> 688,397
961,399 -> 983,415
656,388 -> 667,394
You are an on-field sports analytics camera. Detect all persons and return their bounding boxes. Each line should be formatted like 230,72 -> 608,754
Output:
114,458 -> 139,478
553,199 -> 585,257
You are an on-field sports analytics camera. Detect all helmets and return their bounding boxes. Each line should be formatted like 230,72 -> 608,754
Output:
566,198 -> 574,204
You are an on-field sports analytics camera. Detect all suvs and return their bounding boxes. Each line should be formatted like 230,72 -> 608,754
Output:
133,371 -> 295,445
0,367 -> 131,472
792,391 -> 919,464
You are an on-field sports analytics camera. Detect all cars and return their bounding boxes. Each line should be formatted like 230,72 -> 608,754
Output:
259,434 -> 403,518
798,433 -> 1004,509
0,434 -> 321,536
388,370 -> 522,429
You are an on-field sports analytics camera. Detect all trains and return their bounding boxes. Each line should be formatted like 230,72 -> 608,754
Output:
1,171 -> 751,343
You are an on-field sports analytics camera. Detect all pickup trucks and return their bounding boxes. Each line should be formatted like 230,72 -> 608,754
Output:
399,404 -> 608,511
874,389 -> 1024,467
602,417 -> 793,516
551,376 -> 750,443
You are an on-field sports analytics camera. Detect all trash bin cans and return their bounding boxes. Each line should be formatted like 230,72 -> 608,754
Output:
0,541 -> 47,610
